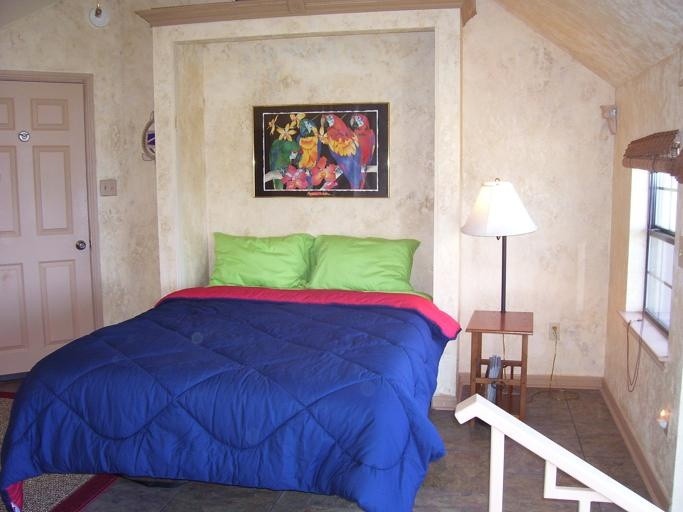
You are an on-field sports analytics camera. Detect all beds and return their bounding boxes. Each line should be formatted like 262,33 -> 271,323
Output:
17,287 -> 433,512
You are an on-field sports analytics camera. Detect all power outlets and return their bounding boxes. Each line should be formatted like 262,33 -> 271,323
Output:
549,322 -> 560,341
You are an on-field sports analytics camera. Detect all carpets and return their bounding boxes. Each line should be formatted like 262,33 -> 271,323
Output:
0,393 -> 119,512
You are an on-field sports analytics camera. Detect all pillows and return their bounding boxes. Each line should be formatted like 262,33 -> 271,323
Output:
207,232 -> 420,292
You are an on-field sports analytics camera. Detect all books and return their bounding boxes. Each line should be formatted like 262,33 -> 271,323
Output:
486,355 -> 503,403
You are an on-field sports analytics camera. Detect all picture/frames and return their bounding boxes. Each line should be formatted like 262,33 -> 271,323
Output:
252,102 -> 390,199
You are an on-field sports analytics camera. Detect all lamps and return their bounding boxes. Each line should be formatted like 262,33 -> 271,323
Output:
600,103 -> 618,133
460,178 -> 537,312
89,0 -> 110,28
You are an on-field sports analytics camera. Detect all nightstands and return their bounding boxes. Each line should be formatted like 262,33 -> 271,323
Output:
465,310 -> 533,422
